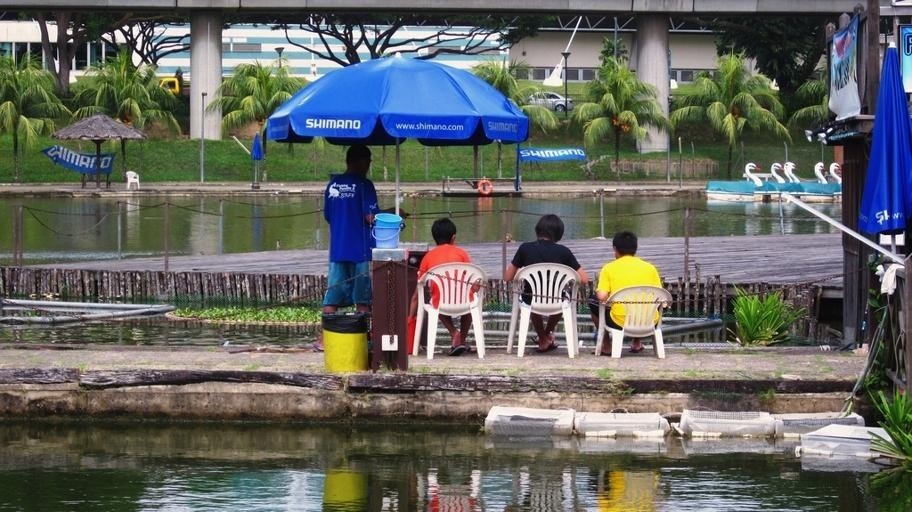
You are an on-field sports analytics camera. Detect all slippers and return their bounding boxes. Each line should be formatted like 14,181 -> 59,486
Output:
314,340 -> 325,351
448,345 -> 466,356
464,345 -> 476,354
592,349 -> 611,356
630,342 -> 644,352
536,339 -> 560,353
531,335 -> 540,345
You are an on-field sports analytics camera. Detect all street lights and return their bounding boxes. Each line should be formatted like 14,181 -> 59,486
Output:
200,91 -> 207,184
275,47 -> 284,68
561,51 -> 572,117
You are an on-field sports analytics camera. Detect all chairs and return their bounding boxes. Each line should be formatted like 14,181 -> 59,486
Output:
412,262 -> 487,361
126,171 -> 140,189
507,455 -> 585,512
506,261 -> 584,359
592,285 -> 674,359
597,467 -> 661,512
413,464 -> 484,512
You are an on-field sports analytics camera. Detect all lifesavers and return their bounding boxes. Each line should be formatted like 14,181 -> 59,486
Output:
478,180 -> 493,195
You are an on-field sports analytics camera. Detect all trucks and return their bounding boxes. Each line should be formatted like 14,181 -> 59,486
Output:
158,77 -> 190,96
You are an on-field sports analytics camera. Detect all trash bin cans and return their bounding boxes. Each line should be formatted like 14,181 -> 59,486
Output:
321,312 -> 370,372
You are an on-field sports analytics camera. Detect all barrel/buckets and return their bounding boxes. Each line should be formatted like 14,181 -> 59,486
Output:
371,226 -> 401,250
372,213 -> 403,228
406,316 -> 416,355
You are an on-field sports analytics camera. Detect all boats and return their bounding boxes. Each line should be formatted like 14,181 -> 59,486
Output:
706,161 -> 842,204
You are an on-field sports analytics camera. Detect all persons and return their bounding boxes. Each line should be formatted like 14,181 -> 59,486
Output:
504,214 -> 588,353
588,231 -> 663,356
315,145 -> 405,348
409,218 -> 480,356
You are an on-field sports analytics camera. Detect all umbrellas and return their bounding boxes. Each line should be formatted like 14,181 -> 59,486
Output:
267,52 -> 529,216
53,113 -> 148,189
857,42 -> 912,264
251,131 -> 264,186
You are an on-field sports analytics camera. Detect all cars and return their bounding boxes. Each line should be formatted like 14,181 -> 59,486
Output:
518,92 -> 574,112
